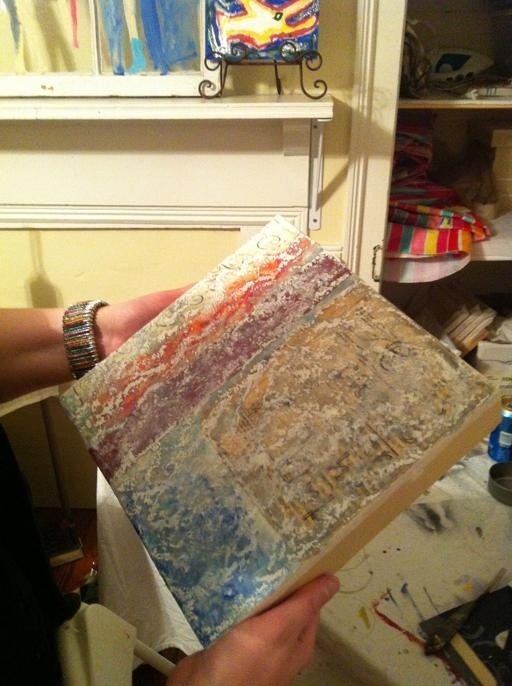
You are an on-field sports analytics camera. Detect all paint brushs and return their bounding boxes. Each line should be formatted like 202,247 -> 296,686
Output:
424,566 -> 508,656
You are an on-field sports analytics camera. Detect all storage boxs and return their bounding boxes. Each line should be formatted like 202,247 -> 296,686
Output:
472,341 -> 512,400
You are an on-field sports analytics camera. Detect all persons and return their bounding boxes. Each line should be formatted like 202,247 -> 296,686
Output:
0,280 -> 343,686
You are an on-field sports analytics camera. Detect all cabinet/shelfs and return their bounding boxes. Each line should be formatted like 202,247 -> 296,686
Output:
397,84 -> 512,264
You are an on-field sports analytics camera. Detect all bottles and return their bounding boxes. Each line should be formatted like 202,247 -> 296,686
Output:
486,394 -> 512,465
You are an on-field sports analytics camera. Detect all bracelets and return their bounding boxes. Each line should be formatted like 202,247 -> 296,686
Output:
63,300 -> 109,382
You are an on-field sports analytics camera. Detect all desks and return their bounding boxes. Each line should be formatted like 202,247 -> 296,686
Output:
319,453 -> 512,684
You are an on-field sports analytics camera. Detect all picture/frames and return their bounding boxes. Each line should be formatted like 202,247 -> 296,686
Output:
0,0 -> 225,99
